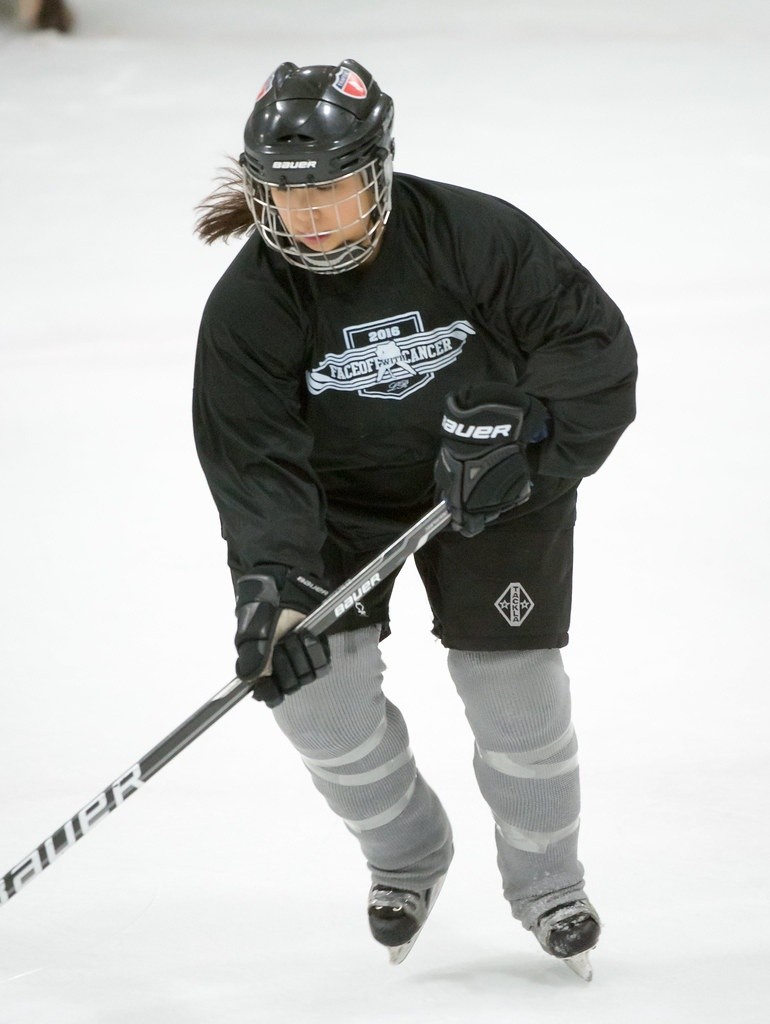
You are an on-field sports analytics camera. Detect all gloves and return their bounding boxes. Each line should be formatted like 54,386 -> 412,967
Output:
436,396 -> 532,536
232,567 -> 330,709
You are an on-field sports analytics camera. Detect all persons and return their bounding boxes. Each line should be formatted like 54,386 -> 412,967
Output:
193,61 -> 639,958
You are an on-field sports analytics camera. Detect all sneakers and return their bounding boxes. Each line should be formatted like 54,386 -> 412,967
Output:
369,884 -> 431,946
535,901 -> 600,956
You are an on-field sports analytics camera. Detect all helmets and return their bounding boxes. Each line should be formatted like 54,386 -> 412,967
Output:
242,59 -> 395,273
